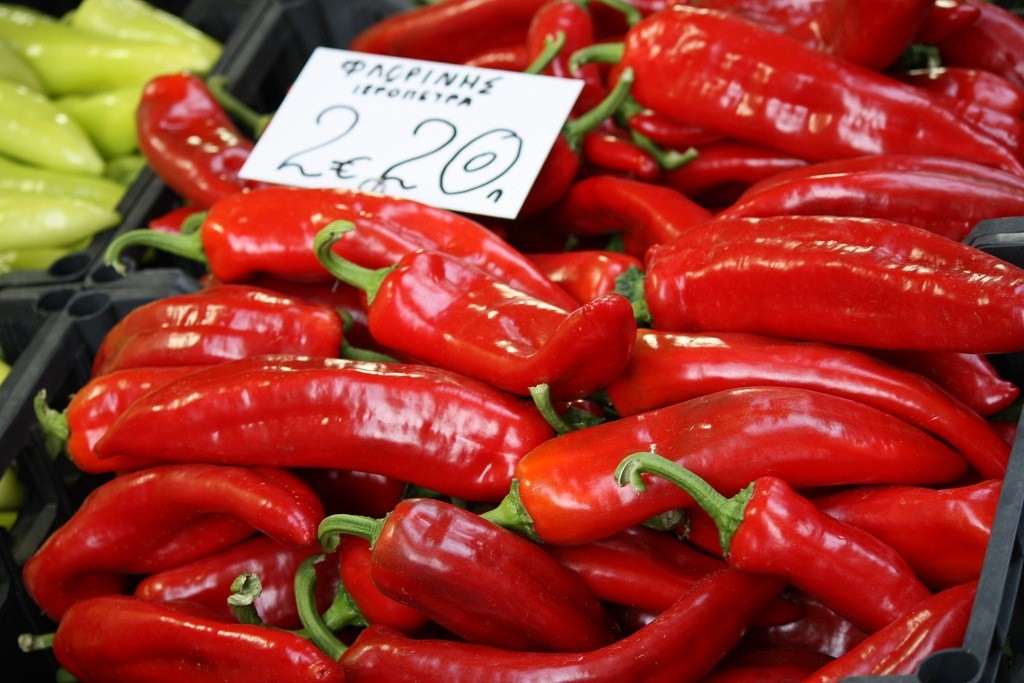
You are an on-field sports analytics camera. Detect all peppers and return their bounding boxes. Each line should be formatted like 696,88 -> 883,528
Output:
0,0 -> 1023,681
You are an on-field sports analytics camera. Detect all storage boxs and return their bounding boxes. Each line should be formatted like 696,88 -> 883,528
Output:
0,1 -> 1024,683
